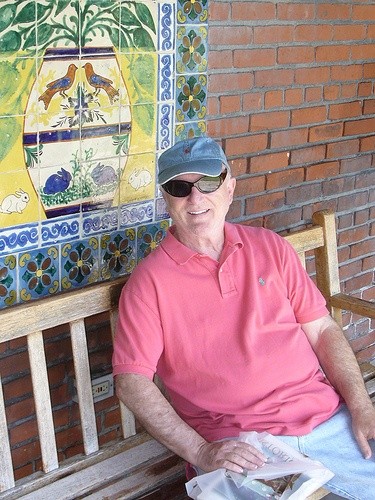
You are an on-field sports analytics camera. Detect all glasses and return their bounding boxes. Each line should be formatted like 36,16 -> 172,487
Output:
161,173 -> 228,198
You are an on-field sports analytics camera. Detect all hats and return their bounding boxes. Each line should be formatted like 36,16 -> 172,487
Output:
157,137 -> 231,186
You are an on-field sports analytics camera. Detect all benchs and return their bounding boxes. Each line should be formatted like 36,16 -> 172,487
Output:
1,210 -> 375,500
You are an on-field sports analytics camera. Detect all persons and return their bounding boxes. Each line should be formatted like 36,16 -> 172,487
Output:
111,136 -> 374,500
